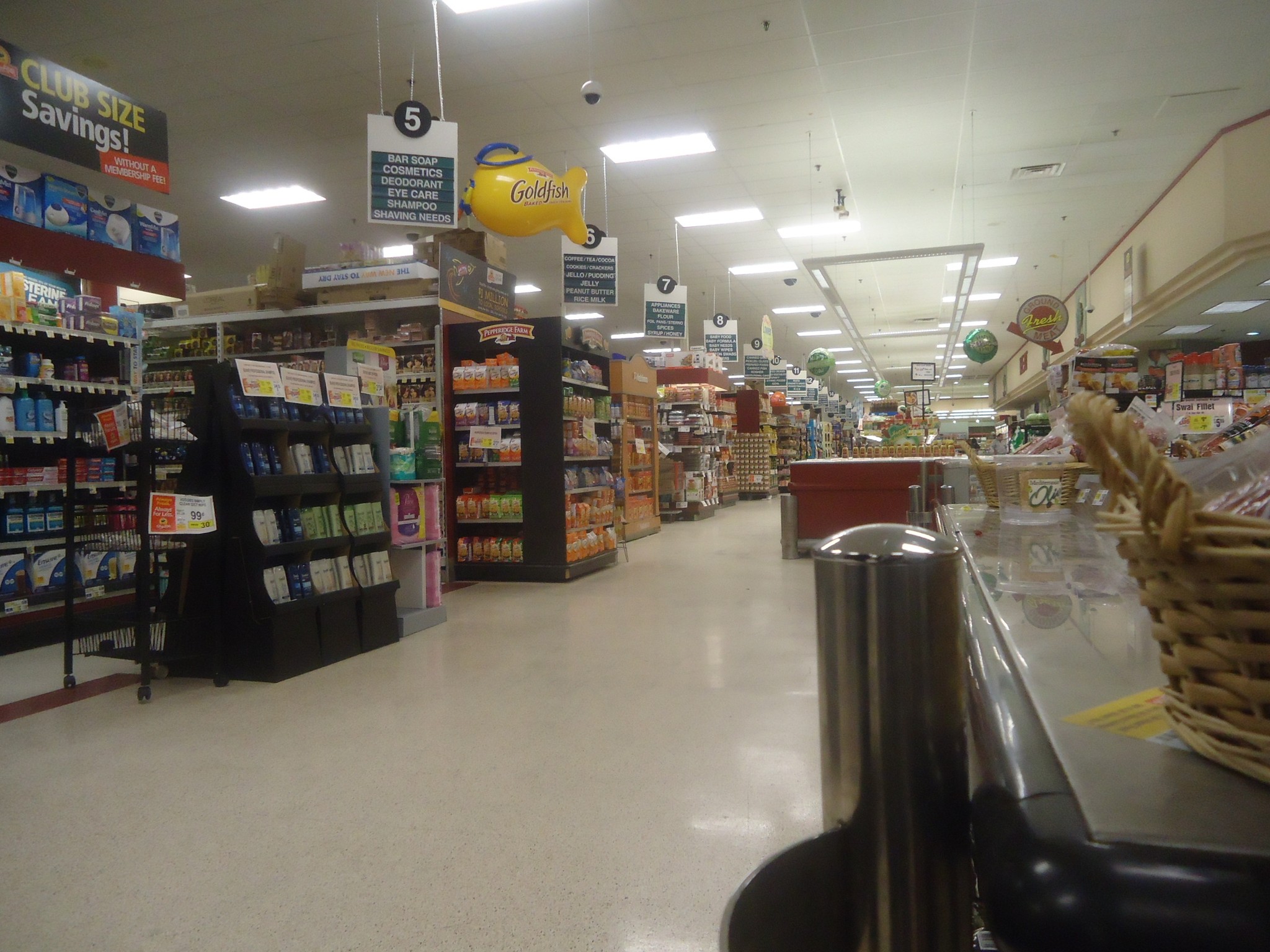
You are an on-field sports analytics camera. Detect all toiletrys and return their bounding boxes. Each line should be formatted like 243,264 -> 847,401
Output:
300,504 -> 343,541
227,379 -> 261,419
0,457 -> 116,486
252,508 -> 303,545
240,442 -> 282,476
273,566 -> 291,604
0,396 -> 15,431
339,501 -> 385,537
310,376 -> 364,424
369,552 -> 384,585
333,444 -> 375,476
298,562 -> 313,598
263,568 -> 279,605
265,397 -> 301,421
14,389 -> 35,431
284,563 -> 303,600
352,554 -> 373,588
310,558 -> 341,594
35,391 -> 55,431
55,400 -> 67,432
336,556 -> 353,589
289,443 -> 331,475
380,550 -> 393,583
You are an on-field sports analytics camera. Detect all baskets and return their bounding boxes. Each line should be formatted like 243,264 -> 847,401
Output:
1065,390 -> 1269,784
960,441 -> 1090,512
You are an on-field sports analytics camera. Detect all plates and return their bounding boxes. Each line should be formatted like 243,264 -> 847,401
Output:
907,429 -> 925,436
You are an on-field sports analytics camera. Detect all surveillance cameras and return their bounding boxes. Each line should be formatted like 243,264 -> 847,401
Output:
580,81 -> 603,105
811,312 -> 821,317
1085,305 -> 1095,313
784,279 -> 797,286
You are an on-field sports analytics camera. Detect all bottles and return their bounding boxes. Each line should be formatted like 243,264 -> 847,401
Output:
940,443 -> 955,456
866,444 -> 874,457
874,444 -> 940,457
859,444 -> 866,457
841,445 -> 849,459
852,445 -> 859,457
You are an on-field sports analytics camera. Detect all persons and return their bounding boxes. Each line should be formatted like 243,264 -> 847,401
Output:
957,438 -> 980,455
992,433 -> 1009,454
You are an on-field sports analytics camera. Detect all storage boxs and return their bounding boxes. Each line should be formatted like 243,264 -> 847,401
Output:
268,235 -> 306,292
42,173 -> 88,237
132,202 -> 179,262
87,189 -> 133,251
715,356 -> 723,371
705,353 -> 715,370
665,350 -> 693,367
693,351 -> 706,368
301,264 -> 438,303
412,241 -> 433,261
432,254 -> 507,272
434,228 -> 507,266
173,284 -> 260,317
0,160 -> 42,228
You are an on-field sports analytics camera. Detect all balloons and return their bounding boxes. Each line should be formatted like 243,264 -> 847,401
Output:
807,348 -> 835,378
457,143 -> 588,244
874,379 -> 891,398
963,328 -> 998,365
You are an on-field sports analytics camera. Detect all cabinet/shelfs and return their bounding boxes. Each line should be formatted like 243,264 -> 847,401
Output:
963,389 -> 1270,462
0,218 -> 963,672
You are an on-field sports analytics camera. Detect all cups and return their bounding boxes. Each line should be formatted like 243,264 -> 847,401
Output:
736,465 -> 769,469
736,433 -> 770,437
735,449 -> 769,452
737,481 -> 768,484
732,444 -> 767,447
737,470 -> 768,474
736,454 -> 768,457
732,438 -> 768,442
737,475 -> 769,479
737,486 -> 769,490
736,460 -> 769,463
